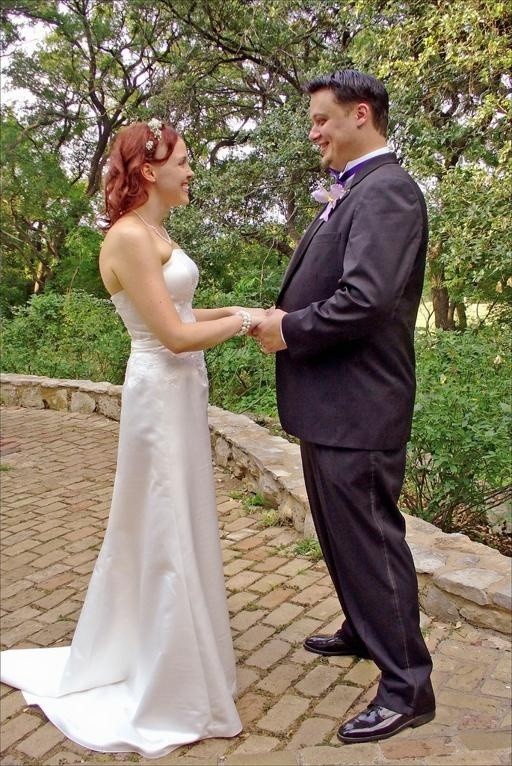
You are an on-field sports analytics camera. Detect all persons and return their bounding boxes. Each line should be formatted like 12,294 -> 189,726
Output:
253,69 -> 437,743
0,119 -> 265,760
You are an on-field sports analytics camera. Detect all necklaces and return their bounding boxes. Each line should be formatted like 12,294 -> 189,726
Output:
130,208 -> 172,246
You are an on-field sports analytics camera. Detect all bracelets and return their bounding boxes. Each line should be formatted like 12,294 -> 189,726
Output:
236,311 -> 251,336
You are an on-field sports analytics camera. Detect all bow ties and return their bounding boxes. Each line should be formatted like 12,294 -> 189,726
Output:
327,170 -> 346,188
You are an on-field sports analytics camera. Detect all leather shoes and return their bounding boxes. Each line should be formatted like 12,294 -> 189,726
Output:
337,702 -> 435,742
303,628 -> 356,656
